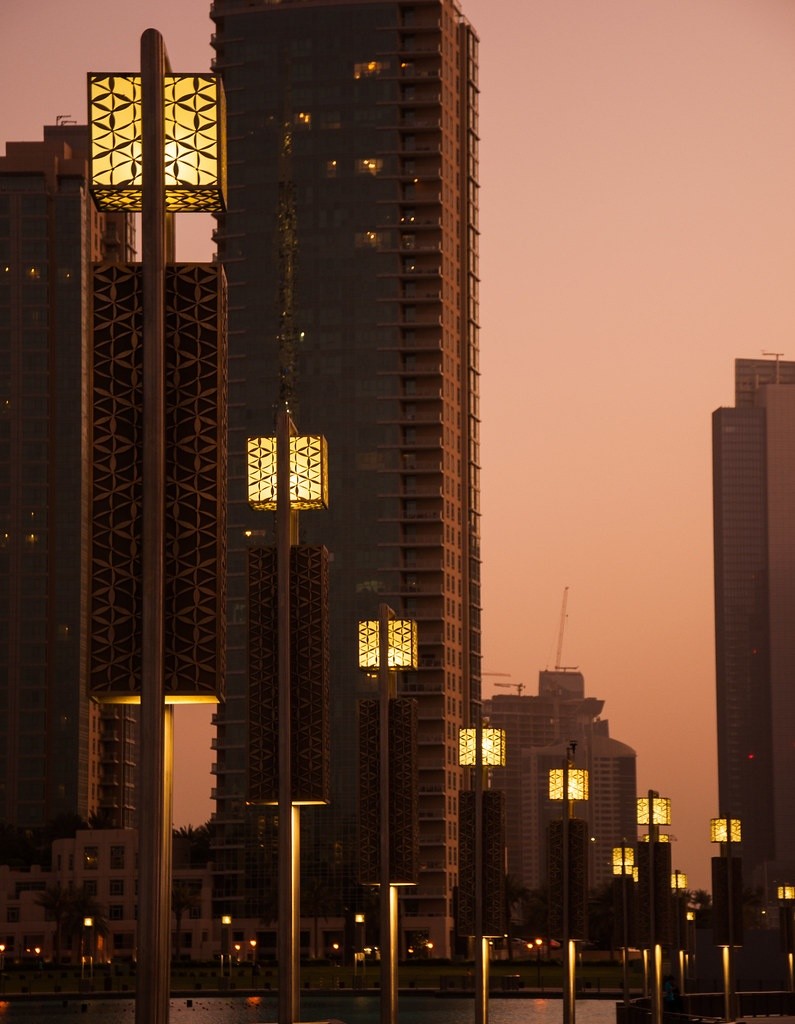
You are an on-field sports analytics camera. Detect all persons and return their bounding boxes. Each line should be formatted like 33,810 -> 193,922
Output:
665,974 -> 684,1013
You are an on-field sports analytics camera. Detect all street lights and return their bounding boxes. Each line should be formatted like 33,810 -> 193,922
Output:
549,752 -> 588,1024
242,395 -> 330,1024
686,911 -> 696,971
635,787 -> 671,1024
529,939 -> 544,987
358,600 -> 419,1023
83,915 -> 92,980
86,25 -> 232,1024
221,912 -> 232,967
613,836 -> 638,1024
458,718 -> 507,1024
671,868 -> 687,997
709,812 -> 742,1024
